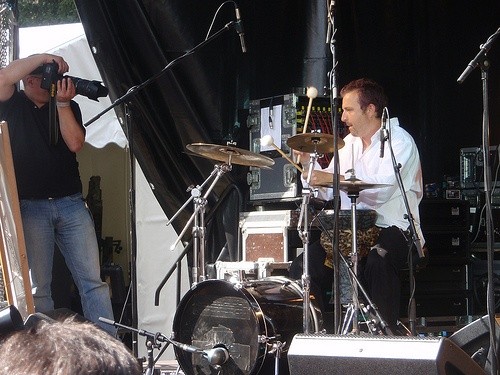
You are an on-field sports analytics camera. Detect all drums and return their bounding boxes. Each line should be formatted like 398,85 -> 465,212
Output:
321,207 -> 379,229
171,275 -> 321,375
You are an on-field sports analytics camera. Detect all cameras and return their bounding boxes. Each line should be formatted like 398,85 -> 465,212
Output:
40,63 -> 109,102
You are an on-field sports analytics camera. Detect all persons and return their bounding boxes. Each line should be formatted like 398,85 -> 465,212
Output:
289,78 -> 424,329
1,54 -> 116,339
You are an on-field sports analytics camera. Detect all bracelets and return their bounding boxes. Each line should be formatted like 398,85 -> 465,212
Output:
57,101 -> 70,107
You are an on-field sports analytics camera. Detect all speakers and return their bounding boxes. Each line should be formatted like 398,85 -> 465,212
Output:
241,226 -> 322,262
447,313 -> 500,375
400,198 -> 474,318
287,332 -> 488,375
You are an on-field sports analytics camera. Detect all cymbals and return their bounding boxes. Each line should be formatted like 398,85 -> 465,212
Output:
185,142 -> 275,167
287,133 -> 344,154
308,177 -> 395,187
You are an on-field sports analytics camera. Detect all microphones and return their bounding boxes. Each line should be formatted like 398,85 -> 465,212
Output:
202,347 -> 229,365
325,0 -> 335,44
379,111 -> 386,158
234,2 -> 247,52
